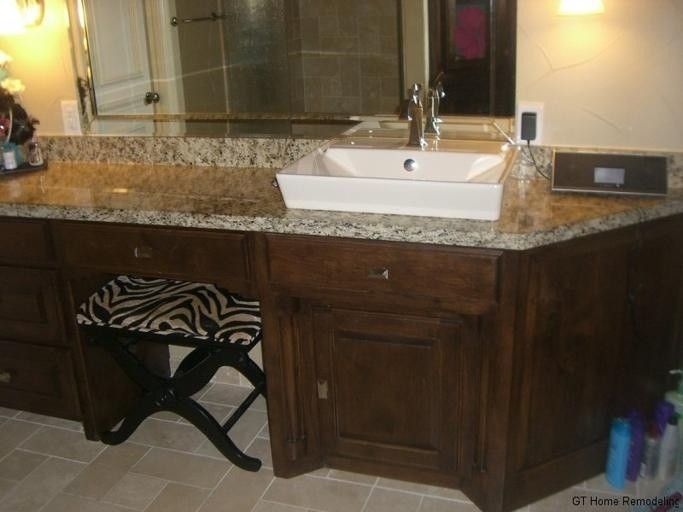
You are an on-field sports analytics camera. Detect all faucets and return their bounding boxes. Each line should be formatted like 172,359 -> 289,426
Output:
423,89 -> 439,134
407,85 -> 426,148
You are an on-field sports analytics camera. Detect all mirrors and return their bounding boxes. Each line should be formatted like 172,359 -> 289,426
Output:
82,0 -> 517,142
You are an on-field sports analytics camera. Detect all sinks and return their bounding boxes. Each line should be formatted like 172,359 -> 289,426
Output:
276,145 -> 519,221
345,116 -> 521,141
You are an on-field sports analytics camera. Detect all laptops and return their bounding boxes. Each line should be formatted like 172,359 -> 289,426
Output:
550,149 -> 670,197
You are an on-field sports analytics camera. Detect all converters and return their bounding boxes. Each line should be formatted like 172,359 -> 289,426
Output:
521,113 -> 537,140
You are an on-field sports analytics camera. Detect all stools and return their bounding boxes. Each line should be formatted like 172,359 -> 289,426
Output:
76,275 -> 266,472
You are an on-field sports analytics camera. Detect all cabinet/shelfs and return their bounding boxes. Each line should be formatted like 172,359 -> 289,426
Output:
254,231 -> 516,512
0,216 -> 171,441
503,212 -> 683,512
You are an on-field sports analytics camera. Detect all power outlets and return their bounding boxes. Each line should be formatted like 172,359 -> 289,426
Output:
515,102 -> 542,145
61,100 -> 83,136
